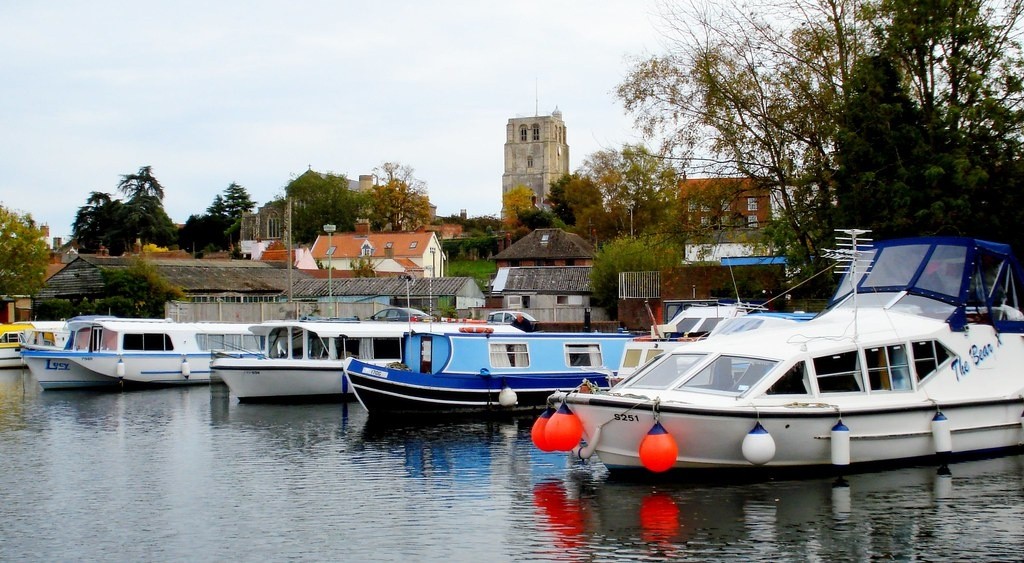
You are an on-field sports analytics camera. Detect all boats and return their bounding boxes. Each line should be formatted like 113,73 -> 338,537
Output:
339,328 -> 637,417
610,299 -> 763,383
0,313 -> 270,391
208,318 -> 532,402
531,234 -> 1024,482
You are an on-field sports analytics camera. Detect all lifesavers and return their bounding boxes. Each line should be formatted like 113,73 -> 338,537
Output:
459,327 -> 495,333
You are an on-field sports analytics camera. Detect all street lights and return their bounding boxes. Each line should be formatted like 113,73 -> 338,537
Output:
324,225 -> 337,320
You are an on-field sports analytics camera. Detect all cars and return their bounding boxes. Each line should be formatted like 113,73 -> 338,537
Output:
486,310 -> 537,327
365,307 -> 438,324
